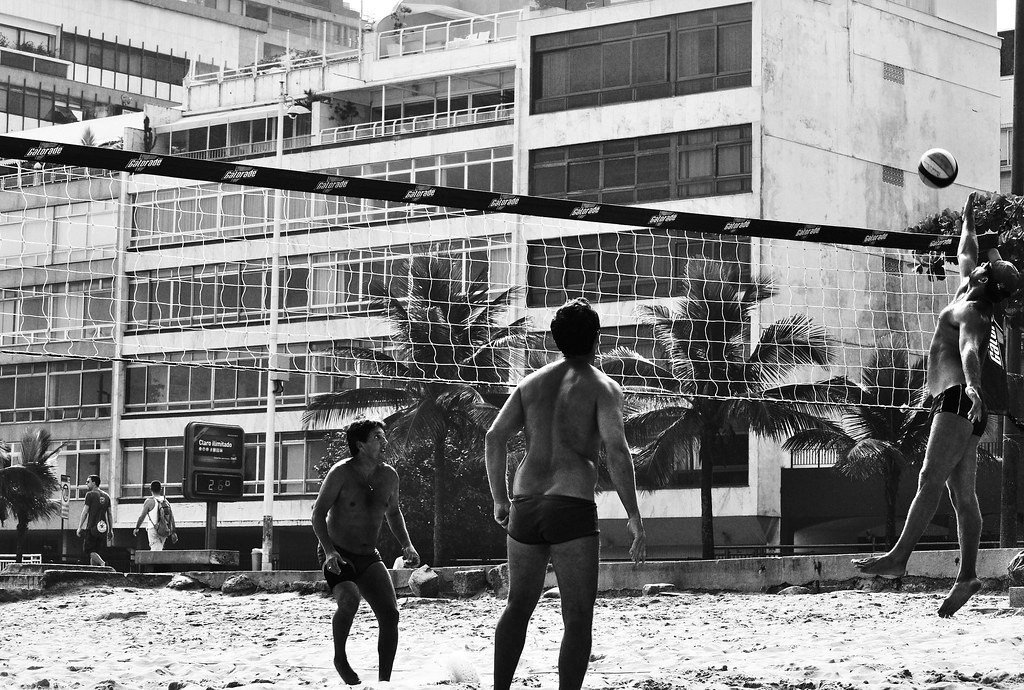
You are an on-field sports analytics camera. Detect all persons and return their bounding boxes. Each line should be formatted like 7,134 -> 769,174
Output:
76,474 -> 114,566
485,296 -> 645,690
852,191 -> 1023,617
310,421 -> 419,685
132,480 -> 178,550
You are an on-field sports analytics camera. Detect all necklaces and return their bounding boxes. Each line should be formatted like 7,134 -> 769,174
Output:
361,471 -> 376,490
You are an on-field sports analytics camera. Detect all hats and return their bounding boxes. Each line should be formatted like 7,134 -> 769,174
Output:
988,248 -> 1020,297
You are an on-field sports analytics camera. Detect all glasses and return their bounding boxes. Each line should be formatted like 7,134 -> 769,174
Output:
983,260 -> 995,285
86,481 -> 89,483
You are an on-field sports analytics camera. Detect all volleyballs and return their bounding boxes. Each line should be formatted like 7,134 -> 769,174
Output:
917,147 -> 959,189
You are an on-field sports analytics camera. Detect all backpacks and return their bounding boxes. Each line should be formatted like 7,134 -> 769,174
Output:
147,496 -> 174,537
